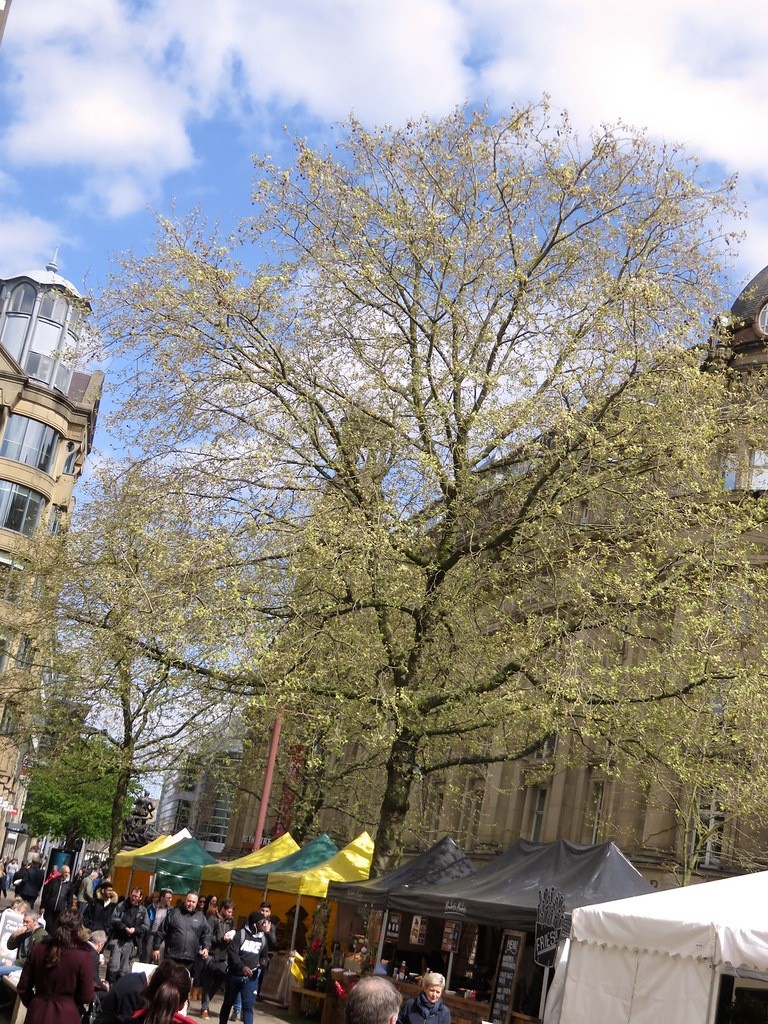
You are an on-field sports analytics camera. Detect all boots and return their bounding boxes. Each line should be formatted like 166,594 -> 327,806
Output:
191,985 -> 203,1001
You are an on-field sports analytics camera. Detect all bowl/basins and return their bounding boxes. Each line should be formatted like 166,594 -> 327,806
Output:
458,987 -> 468,994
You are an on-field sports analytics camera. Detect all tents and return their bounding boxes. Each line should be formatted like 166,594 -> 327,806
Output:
109,828 -> 768,1024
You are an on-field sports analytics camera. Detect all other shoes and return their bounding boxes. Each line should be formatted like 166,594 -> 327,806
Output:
230,1011 -> 240,1021
201,1010 -> 209,1019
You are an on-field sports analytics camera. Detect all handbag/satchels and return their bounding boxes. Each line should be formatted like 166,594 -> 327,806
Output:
37,913 -> 46,930
207,949 -> 230,981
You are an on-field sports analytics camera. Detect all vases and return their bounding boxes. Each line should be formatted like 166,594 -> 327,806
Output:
317,982 -> 331,993
304,979 -> 317,990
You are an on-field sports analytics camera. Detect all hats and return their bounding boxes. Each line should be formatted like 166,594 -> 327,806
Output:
248,912 -> 264,926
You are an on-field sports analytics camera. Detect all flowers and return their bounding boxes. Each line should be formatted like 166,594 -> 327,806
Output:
319,967 -> 332,983
302,937 -> 321,981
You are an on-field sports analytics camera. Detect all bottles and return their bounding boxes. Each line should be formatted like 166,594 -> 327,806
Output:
398,960 -> 406,981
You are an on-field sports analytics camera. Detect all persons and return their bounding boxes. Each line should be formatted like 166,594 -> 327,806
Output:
125,791 -> 157,828
0,852 -> 451,1024
